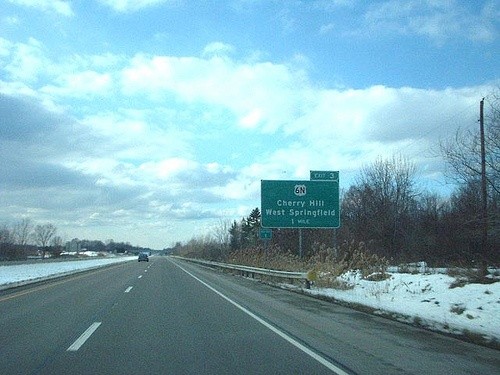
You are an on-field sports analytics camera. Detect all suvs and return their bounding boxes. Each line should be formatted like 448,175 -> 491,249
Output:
138,254 -> 148,263
